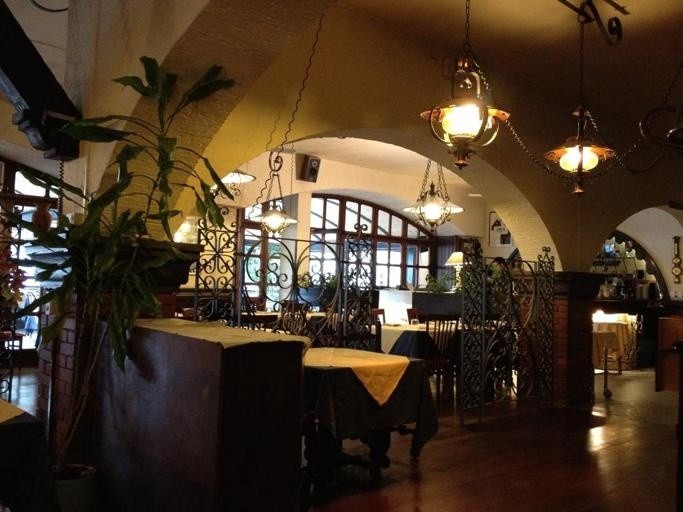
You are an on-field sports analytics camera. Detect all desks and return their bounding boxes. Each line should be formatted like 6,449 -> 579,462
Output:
241,309 -> 355,346
592,330 -> 622,400
95,317 -> 313,512
366,317 -> 507,402
0,397 -> 60,511
301,346 -> 439,485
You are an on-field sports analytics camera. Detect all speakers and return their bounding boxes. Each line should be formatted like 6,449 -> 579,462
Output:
300,154 -> 321,183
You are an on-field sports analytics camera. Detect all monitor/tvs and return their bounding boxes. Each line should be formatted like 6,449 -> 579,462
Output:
592,240 -> 621,267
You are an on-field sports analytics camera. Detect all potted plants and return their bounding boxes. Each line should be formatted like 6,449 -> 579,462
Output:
298,271 -> 336,306
1,57 -> 237,511
459,237 -> 503,293
413,271 -> 466,314
66,204 -> 205,294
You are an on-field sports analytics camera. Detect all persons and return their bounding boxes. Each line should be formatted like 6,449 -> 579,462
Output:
493,257 -> 506,271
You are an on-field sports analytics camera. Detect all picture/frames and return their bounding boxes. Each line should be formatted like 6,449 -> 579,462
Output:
489,211 -> 513,248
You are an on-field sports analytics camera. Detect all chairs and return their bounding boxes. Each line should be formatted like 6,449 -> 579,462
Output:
406,308 -> 424,324
371,308 -> 385,325
424,315 -> 460,410
280,300 -> 304,313
245,296 -> 267,311
181,305 -> 205,317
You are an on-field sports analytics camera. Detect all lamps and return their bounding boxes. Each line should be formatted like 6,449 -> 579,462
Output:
420,0 -> 512,170
541,24 -> 619,199
445,252 -> 471,288
255,169 -> 299,234
221,167 -> 256,197
402,159 -> 464,235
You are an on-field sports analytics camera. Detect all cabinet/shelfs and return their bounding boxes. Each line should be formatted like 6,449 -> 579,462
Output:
593,256 -> 636,301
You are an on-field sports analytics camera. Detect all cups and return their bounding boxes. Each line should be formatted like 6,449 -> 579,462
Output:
411,319 -> 419,330
